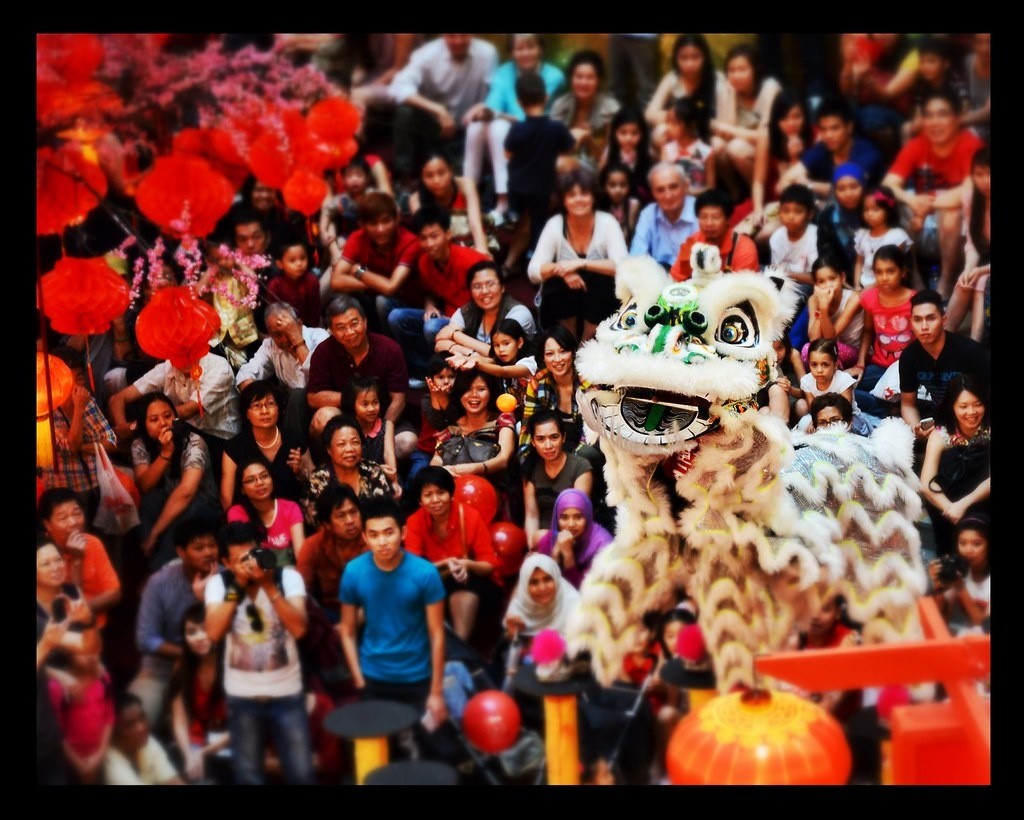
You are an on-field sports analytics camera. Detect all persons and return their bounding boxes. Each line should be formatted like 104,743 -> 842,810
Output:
36,34 -> 990,785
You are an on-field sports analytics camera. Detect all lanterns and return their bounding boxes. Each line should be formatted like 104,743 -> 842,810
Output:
36,352 -> 74,467
667,687 -> 852,784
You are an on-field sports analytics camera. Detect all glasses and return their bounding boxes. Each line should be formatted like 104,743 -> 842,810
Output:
247,603 -> 264,632
242,471 -> 270,485
249,398 -> 278,410
471,279 -> 500,292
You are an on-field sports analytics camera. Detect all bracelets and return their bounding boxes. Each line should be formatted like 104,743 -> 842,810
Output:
82,616 -> 96,629
291,339 -> 305,352
355,267 -> 365,279
270,591 -> 281,602
451,329 -> 460,340
160,453 -> 170,461
468,350 -> 476,357
483,462 -> 488,473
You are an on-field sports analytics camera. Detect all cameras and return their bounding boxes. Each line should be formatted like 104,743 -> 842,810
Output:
251,546 -> 278,571
164,418 -> 189,449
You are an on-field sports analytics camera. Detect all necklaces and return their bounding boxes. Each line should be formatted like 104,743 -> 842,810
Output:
256,425 -> 279,450
483,316 -> 490,342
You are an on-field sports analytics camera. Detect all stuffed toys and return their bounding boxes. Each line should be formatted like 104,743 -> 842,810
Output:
534,258 -> 927,696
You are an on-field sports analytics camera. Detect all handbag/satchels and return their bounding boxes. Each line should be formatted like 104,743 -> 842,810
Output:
436,435 -> 501,466
928,444 -> 991,503
93,441 -> 141,534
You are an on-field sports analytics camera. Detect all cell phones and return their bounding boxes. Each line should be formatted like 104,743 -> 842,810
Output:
51,597 -> 67,623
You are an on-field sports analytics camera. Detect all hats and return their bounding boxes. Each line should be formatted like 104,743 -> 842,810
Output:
557,493 -> 587,518
833,163 -> 865,183
